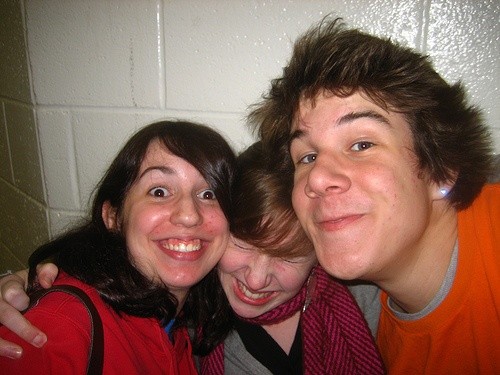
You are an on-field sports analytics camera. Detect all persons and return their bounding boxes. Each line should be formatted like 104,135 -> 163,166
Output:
0,134 -> 388,375
240,10 -> 500,375
0,118 -> 243,375
